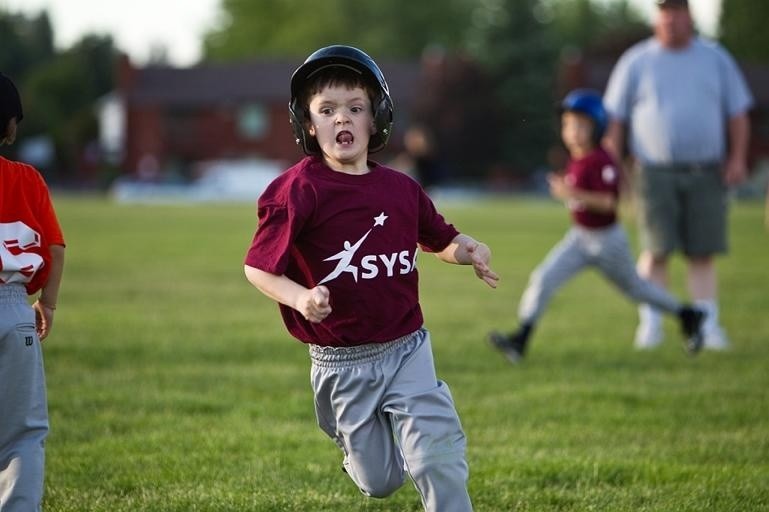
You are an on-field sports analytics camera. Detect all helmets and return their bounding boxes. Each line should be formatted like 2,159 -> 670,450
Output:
557,89 -> 607,144
287,45 -> 394,156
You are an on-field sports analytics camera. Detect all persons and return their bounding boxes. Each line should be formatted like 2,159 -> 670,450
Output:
241,43 -> 503,512
1,72 -> 67,510
488,88 -> 713,365
599,0 -> 758,354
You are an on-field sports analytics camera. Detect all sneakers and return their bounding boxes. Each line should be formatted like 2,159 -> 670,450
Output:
632,323 -> 664,351
680,305 -> 707,354
487,332 -> 526,366
700,321 -> 729,352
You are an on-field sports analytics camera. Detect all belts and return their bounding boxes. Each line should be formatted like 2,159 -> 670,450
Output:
635,155 -> 723,177
